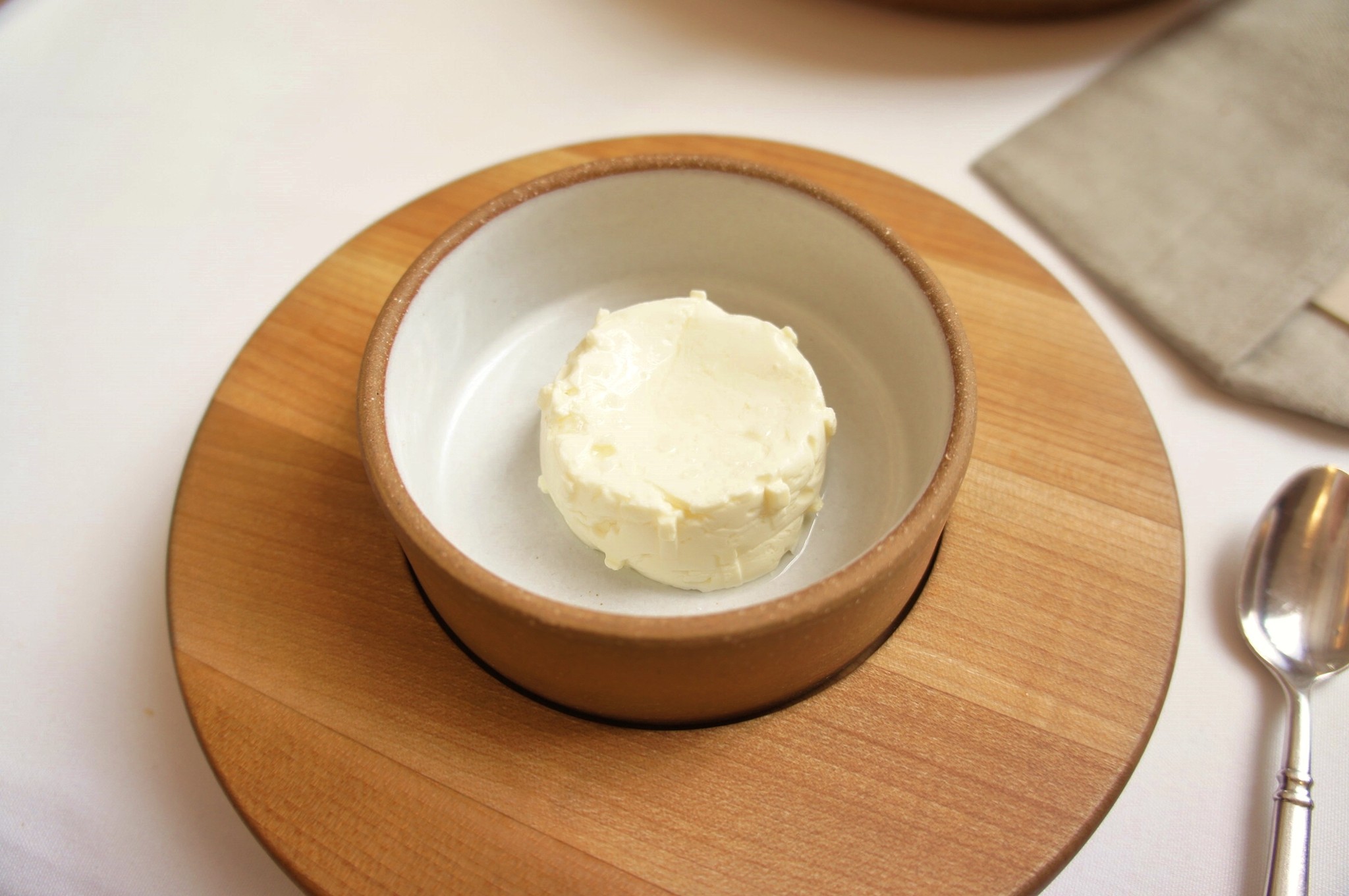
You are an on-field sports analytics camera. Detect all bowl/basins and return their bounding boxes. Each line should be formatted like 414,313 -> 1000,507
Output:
355,150 -> 975,728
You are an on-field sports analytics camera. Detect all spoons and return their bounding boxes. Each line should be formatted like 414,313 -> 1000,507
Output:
1240,466 -> 1349,896
